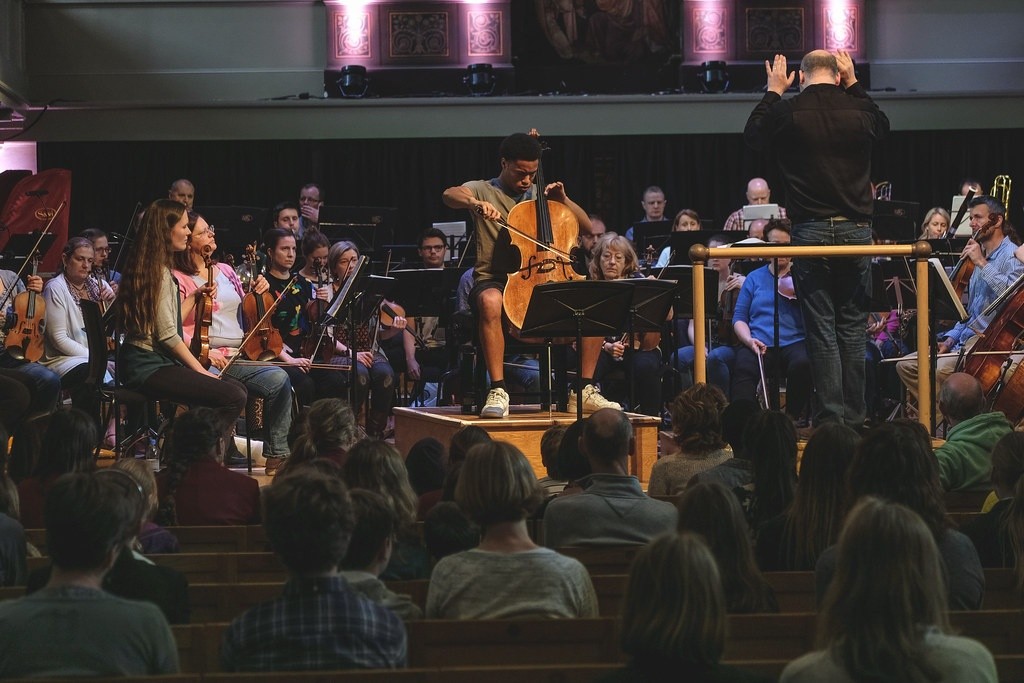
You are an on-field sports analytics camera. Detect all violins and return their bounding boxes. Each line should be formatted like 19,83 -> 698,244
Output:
88,261 -> 116,350
240,244 -> 283,361
954,211 -> 1000,300
2,249 -> 46,363
189,244 -> 218,371
376,299 -> 429,352
625,244 -> 661,351
298,257 -> 333,365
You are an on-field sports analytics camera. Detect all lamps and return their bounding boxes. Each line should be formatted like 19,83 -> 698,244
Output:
337,65 -> 370,98
697,59 -> 730,93
464,64 -> 496,95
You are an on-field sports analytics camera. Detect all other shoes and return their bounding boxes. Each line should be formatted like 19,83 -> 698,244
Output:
264,459 -> 280,476
794,426 -> 814,440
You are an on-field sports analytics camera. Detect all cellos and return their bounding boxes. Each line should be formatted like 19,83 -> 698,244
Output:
501,128 -> 588,419
934,280 -> 1024,429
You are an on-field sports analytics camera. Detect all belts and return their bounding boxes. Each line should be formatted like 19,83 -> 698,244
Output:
792,215 -> 871,223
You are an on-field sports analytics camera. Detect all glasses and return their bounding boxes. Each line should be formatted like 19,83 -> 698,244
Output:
421,245 -> 445,252
191,225 -> 215,237
95,246 -> 112,253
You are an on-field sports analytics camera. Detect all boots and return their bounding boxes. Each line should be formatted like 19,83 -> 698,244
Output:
223,436 -> 257,469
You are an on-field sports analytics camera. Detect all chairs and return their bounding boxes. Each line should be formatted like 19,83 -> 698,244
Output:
476,333 -> 572,418
64,301 -> 402,473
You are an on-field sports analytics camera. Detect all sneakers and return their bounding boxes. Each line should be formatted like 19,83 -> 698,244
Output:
567,384 -> 622,414
479,387 -> 509,418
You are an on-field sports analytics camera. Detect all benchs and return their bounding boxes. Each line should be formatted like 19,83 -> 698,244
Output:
0,493 -> 1024,683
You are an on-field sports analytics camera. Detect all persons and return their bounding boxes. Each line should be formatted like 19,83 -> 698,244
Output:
424,440 -> 601,620
156,408 -> 261,526
220,468 -> 409,671
113,456 -> 179,554
0,132 -> 1024,475
336,488 -> 422,620
779,497 -> 999,683
273,398 -> 493,578
935,373 -> 1014,491
0,475 -> 180,677
756,423 -> 863,569
813,418 -> 985,610
677,483 -> 779,612
0,408 -> 98,586
648,382 -> 801,568
744,49 -> 891,439
26,469 -> 189,624
526,407 -> 678,547
973,431 -> 1024,590
592,533 -> 779,683
